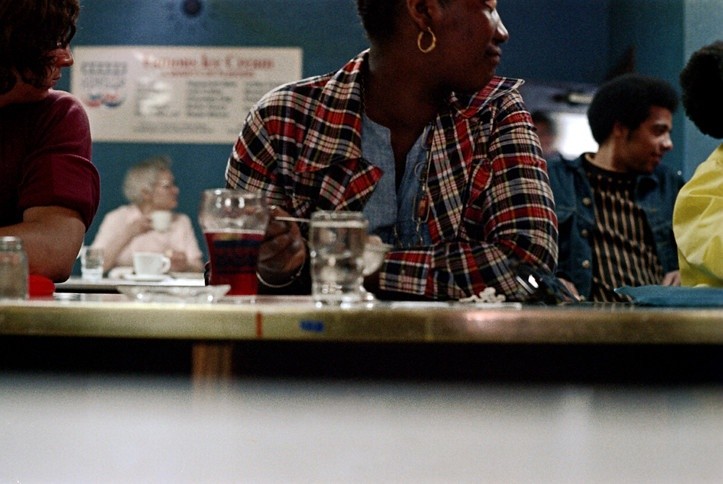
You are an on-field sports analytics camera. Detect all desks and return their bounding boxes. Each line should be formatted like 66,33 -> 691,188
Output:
53,271 -> 206,289
0,292 -> 723,420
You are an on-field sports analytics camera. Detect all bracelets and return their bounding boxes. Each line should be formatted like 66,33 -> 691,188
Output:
256,265 -> 304,288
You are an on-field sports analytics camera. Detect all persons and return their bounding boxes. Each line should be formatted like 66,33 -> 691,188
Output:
673,39 -> 723,289
531,109 -> 556,157
91,155 -> 205,273
0,0 -> 100,284
546,73 -> 687,302
204,0 -> 560,302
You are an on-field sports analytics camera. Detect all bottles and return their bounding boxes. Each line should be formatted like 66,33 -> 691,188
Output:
0,235 -> 29,300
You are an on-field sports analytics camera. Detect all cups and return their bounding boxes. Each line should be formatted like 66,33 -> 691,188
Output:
80,246 -> 105,282
145,210 -> 172,231
308,210 -> 369,309
199,188 -> 269,304
132,252 -> 172,277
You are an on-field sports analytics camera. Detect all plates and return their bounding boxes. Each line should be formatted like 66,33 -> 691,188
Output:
125,274 -> 173,282
119,287 -> 229,303
